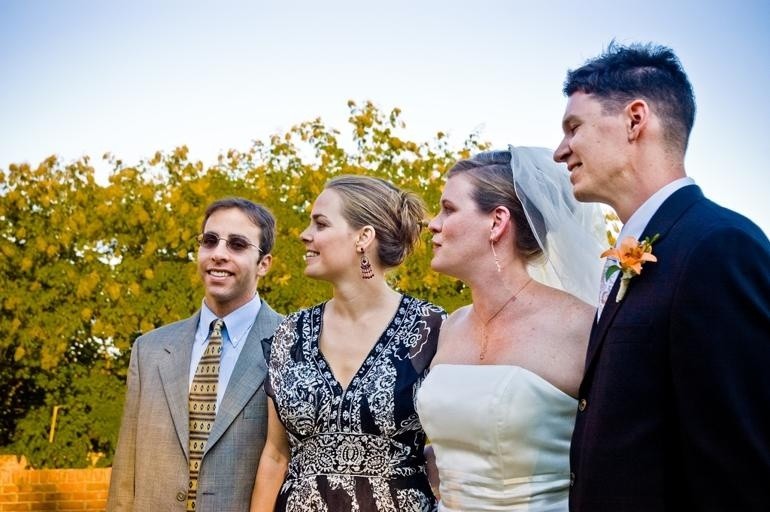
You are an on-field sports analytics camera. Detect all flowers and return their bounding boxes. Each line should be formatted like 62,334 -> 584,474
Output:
594,229 -> 664,303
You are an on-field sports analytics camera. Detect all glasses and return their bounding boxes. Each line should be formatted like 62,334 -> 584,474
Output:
196,232 -> 263,255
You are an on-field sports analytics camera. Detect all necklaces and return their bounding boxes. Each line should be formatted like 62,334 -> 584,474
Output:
474,277 -> 534,359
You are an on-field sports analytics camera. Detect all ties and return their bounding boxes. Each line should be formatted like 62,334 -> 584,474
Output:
598,248 -> 620,321
186,319 -> 225,512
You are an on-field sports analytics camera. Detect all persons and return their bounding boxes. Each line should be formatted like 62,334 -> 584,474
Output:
414,142 -> 614,511
553,36 -> 770,512
250,175 -> 449,511
105,195 -> 289,512
